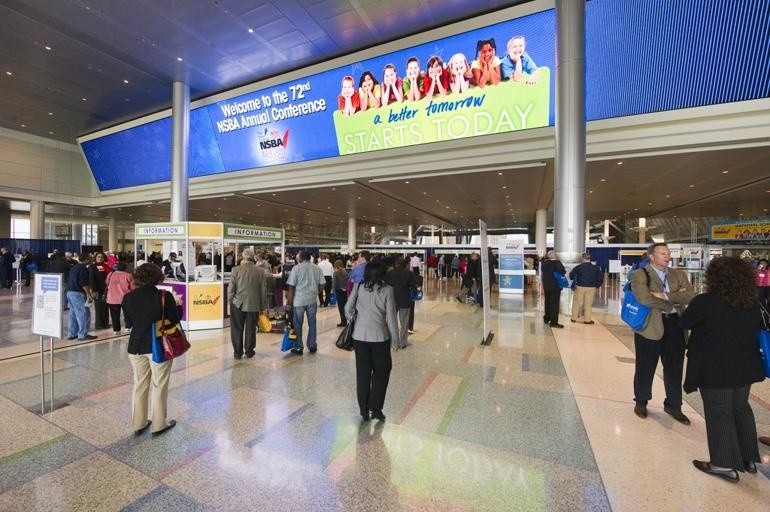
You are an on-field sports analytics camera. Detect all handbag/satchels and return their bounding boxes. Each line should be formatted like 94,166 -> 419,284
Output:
280,327 -> 297,352
571,276 -> 578,291
410,289 -> 422,301
757,327 -> 770,377
27,263 -> 37,272
152,319 -> 191,364
554,272 -> 569,288
335,320 -> 355,351
102,286 -> 108,300
330,293 -> 336,305
620,290 -> 650,332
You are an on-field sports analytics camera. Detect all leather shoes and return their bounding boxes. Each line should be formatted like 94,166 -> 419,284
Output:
363,411 -> 385,421
79,335 -> 97,340
152,419 -> 176,436
663,406 -> 691,424
634,403 -> 650,418
693,459 -> 739,482
135,419 -> 153,435
744,460 -> 758,473
68,335 -> 78,340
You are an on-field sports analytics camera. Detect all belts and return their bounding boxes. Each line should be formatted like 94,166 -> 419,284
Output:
661,312 -> 680,319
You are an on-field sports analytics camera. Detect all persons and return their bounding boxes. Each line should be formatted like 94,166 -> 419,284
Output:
733,223 -> 769,241
1,244 -> 244,341
681,259 -> 766,483
123,262 -> 181,434
337,35 -> 539,116
569,254 -> 603,325
632,242 -> 693,427
346,259 -> 402,422
629,251 -> 650,272
539,251 -> 564,330
253,246 -> 535,358
754,257 -> 770,329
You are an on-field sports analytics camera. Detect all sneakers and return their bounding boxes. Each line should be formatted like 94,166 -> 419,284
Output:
125,327 -> 133,334
543,315 -> 564,328
291,349 -> 303,355
571,318 -> 576,322
584,320 -> 594,324
309,347 -> 317,353
337,323 -> 347,326
759,436 -> 769,445
407,329 -> 415,334
234,351 -> 255,359
113,331 -> 121,336
